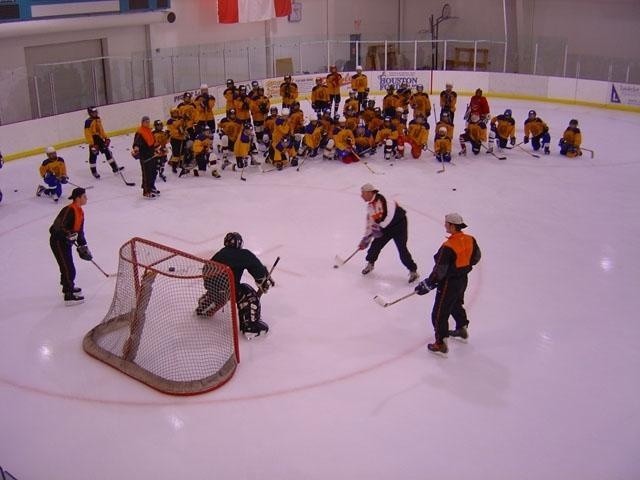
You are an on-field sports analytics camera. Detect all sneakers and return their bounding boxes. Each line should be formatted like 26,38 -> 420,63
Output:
64,293 -> 84,301
212,170 -> 221,178
449,325 -> 469,339
408,271 -> 419,283
244,319 -> 269,332
73,287 -> 81,293
112,166 -> 124,173
428,338 -> 449,353
143,191 -> 156,198
37,185 -> 46,197
92,170 -> 100,178
52,193 -> 58,200
152,188 -> 160,194
362,263 -> 374,274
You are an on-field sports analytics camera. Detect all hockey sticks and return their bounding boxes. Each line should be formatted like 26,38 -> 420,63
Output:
57,178 -> 93,190
506,138 -> 531,149
296,154 -> 309,172
374,290 -> 418,308
582,148 -> 594,159
482,143 -> 507,160
437,154 -> 445,173
240,161 -> 246,182
515,142 -> 540,159
350,149 -> 386,176
74,242 -> 121,277
109,148 -> 136,186
335,232 -> 374,264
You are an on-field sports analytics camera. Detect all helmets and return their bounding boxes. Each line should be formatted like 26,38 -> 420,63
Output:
223,232 -> 243,249
330,65 -> 337,73
45,146 -> 56,155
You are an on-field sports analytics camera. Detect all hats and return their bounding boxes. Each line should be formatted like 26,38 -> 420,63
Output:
356,66 -> 363,70
445,213 -> 468,229
227,79 -> 234,84
68,188 -> 86,200
200,84 -> 208,89
360,183 -> 379,193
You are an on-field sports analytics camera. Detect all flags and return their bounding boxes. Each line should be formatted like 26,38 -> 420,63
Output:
218,0 -> 292,24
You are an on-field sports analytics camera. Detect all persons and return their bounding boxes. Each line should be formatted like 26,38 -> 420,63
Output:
289,102 -> 304,132
193,84 -> 216,135
266,106 -> 279,132
280,74 -> 298,108
359,99 -> 375,125
336,123 -> 360,163
435,127 -> 451,162
396,82 -> 412,121
234,84 -> 253,123
252,87 -> 270,125
486,109 -> 517,154
326,65 -> 343,115
255,131 -> 272,166
458,111 -> 487,156
345,106 -> 358,132
37,146 -> 69,201
312,77 -> 331,117
351,66 -> 369,109
396,112 -> 430,160
132,116 -> 161,200
193,125 -> 222,180
378,117 -> 397,159
414,213 -> 481,356
559,119 -> 583,158
231,124 -> 254,183
224,79 -> 239,119
167,108 -> 188,173
217,108 -> 240,166
305,114 -> 321,158
465,88 -> 490,124
354,119 -> 376,156
344,91 -> 361,124
440,82 -> 457,117
151,120 -> 168,182
49,188 -> 93,306
411,85 -> 431,121
320,108 -> 333,147
84,105 -> 125,181
272,108 -> 298,170
248,80 -> 259,96
524,109 -> 550,155
196,232 -> 274,341
360,183 -> 419,286
436,111 -> 454,141
393,107 -> 407,134
177,93 -> 196,164
383,85 -> 399,118
